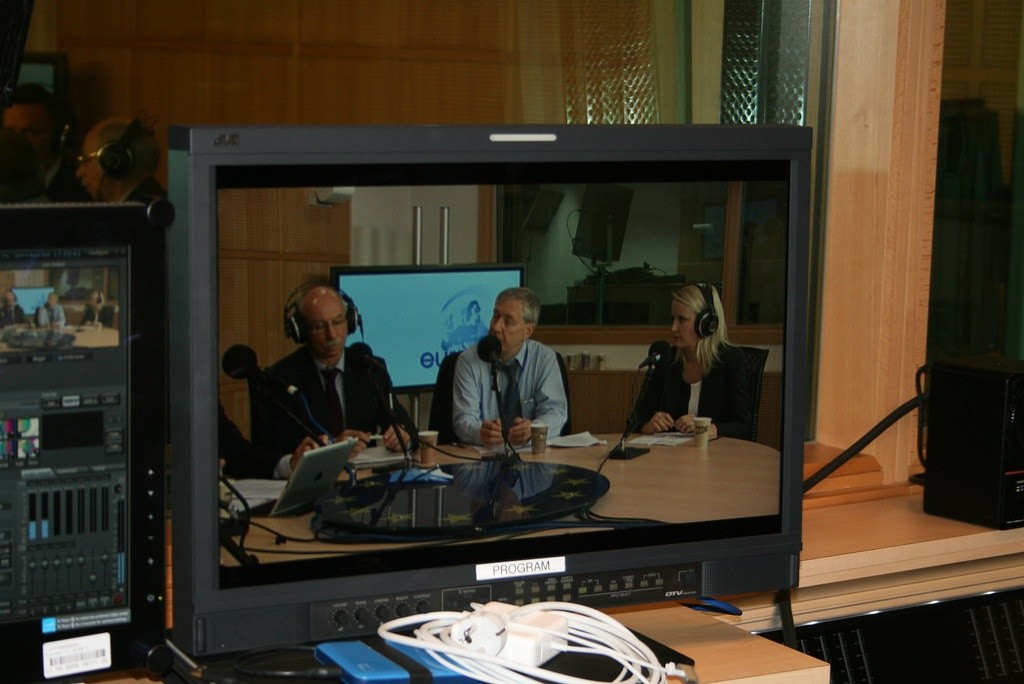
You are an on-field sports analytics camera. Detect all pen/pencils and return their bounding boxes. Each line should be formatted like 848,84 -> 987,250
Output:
368,435 -> 383,440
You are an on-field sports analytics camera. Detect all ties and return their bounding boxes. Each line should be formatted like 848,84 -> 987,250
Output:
502,361 -> 524,441
320,367 -> 342,438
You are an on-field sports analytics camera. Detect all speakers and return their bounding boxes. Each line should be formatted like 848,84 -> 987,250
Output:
569,179 -> 633,263
519,187 -> 565,236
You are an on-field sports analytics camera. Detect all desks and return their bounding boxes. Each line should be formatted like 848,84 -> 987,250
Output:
66,598 -> 830,684
0,325 -> 119,353
566,282 -> 690,325
221,432 -> 781,569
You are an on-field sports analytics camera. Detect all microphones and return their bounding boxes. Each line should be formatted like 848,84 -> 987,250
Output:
222,344 -> 300,397
639,340 -> 671,369
477,336 -> 502,389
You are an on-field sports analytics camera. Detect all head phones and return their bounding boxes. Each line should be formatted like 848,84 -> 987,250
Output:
97,118 -> 152,179
3,289 -> 17,304
12,83 -> 77,153
95,290 -> 103,304
693,280 -> 719,338
282,279 -> 359,344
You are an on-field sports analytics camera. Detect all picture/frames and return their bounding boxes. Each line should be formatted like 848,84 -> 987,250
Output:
701,202 -> 726,259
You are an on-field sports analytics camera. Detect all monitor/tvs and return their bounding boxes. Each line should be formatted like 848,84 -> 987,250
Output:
165,123 -> 815,659
331,262 -> 525,395
11,286 -> 55,315
0,191 -> 175,684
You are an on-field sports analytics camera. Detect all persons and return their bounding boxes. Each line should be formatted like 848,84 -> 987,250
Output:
80,290 -> 112,328
0,291 -> 26,329
0,83 -> 171,204
219,394 -> 329,479
248,286 -> 419,453
454,287 -> 569,446
38,292 -> 66,328
633,282 -> 755,442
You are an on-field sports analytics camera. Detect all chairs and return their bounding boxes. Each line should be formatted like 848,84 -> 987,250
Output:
741,345 -> 769,442
426,349 -> 572,446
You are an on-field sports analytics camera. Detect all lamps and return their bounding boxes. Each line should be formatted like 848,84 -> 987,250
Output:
308,186 -> 355,208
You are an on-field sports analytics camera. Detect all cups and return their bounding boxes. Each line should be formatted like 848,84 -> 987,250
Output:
530,424 -> 548,454
694,416 -> 712,448
596,355 -> 605,370
567,354 -> 576,370
418,431 -> 438,464
581,351 -> 591,370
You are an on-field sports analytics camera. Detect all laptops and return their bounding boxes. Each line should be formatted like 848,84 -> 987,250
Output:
0,324 -> 18,343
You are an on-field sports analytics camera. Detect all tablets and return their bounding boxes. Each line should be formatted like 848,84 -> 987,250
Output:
271,437 -> 358,517
